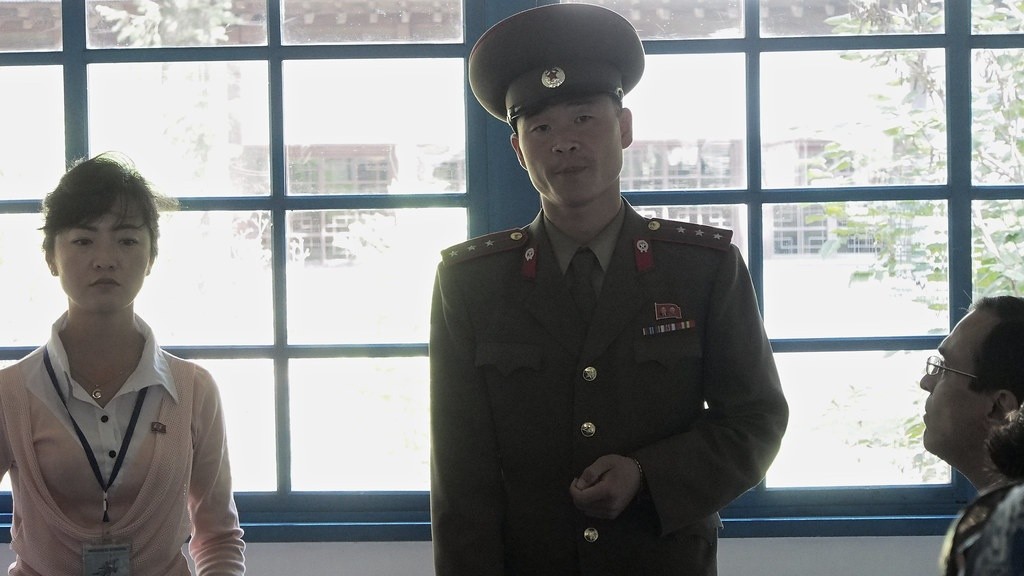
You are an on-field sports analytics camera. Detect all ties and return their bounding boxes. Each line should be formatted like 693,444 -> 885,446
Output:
565,249 -> 596,324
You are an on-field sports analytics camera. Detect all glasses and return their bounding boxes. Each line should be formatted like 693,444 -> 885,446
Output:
926,355 -> 988,386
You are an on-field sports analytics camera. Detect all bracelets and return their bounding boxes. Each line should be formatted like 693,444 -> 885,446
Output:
633,457 -> 648,504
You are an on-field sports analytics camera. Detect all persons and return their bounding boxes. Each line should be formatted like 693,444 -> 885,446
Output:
920,296 -> 1024,576
0,157 -> 245,576
431,3 -> 789,576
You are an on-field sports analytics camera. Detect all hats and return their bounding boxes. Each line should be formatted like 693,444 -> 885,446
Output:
466,4 -> 644,133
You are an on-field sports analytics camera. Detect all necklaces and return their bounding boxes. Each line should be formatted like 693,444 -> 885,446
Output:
69,365 -> 135,399
